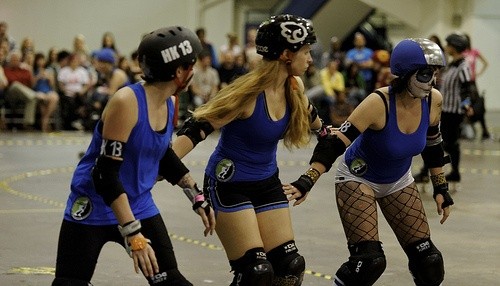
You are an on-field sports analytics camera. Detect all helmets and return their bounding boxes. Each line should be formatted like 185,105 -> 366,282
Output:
97,48 -> 116,64
445,33 -> 471,52
389,38 -> 447,76
137,26 -> 203,82
255,14 -> 318,59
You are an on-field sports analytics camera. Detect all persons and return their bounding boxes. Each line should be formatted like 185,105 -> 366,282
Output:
281,38 -> 454,286
157,17 -> 338,286
0,22 -> 490,182
51,25 -> 216,286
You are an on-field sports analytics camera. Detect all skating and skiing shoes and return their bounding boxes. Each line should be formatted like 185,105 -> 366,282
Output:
413,170 -> 430,194
444,169 -> 462,192
